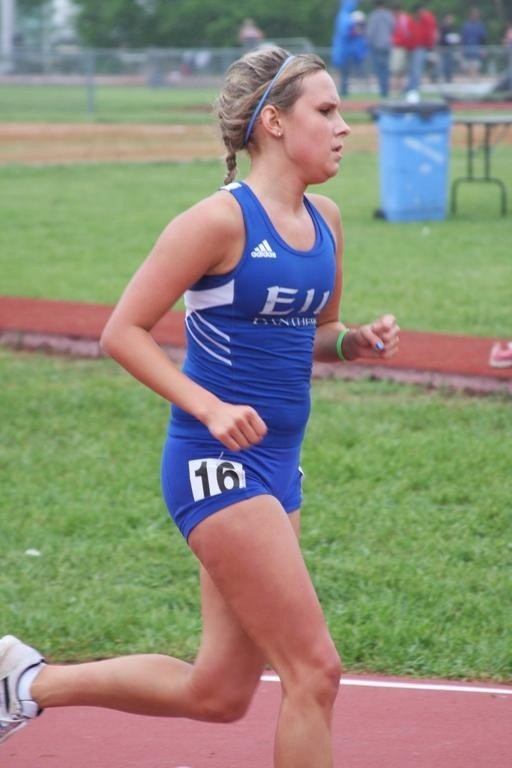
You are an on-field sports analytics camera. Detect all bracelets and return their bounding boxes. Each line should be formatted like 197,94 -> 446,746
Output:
336,328 -> 350,363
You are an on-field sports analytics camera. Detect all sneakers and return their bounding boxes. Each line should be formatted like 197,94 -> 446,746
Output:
0,635 -> 46,741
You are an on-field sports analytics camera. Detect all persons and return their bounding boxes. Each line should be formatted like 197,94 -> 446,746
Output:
330,1 -> 511,98
0,44 -> 400,761
238,18 -> 265,57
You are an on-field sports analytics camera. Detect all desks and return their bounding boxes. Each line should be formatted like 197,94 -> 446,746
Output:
450,115 -> 512,218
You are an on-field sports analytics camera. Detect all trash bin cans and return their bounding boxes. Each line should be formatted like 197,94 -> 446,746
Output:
367,101 -> 451,223
